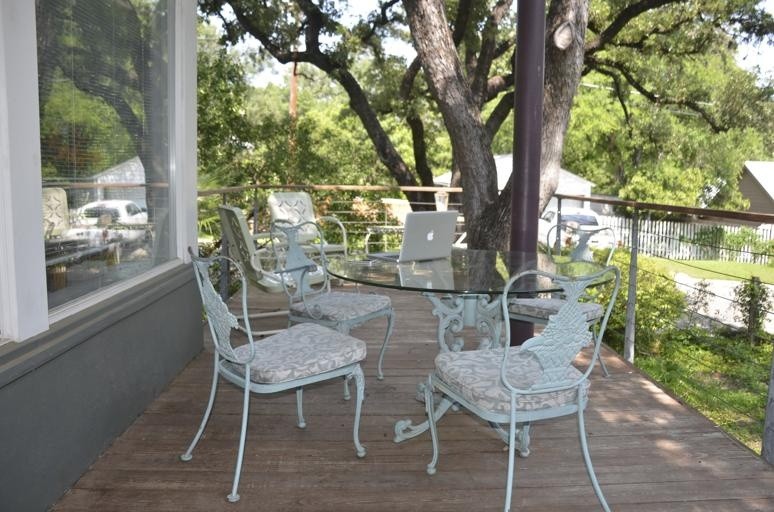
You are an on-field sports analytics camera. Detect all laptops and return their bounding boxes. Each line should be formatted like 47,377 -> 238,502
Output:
366,210 -> 458,262
399,258 -> 454,290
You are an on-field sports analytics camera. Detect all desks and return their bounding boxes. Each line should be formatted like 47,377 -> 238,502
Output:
325,246 -> 619,457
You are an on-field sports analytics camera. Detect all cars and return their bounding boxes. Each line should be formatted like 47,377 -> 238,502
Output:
68,199 -> 148,241
538,208 -> 607,248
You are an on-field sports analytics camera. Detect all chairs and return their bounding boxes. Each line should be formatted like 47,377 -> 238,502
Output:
216,202 -> 332,338
421,263 -> 619,511
266,191 -> 349,294
500,224 -> 617,378
181,245 -> 371,505
271,216 -> 396,383
